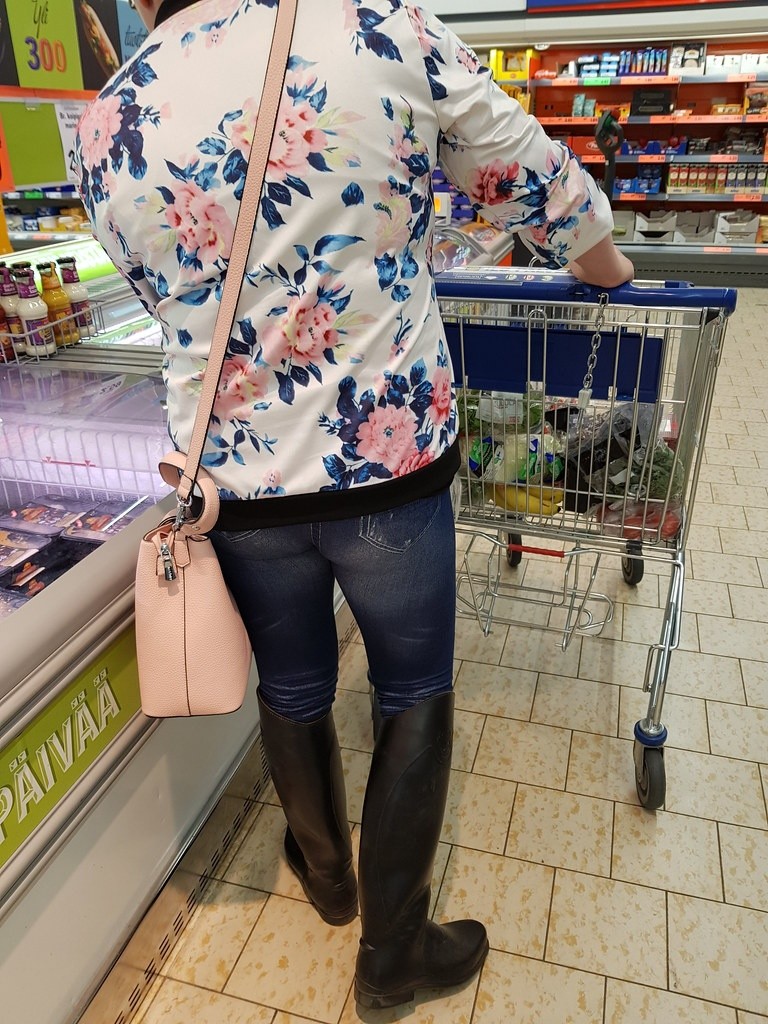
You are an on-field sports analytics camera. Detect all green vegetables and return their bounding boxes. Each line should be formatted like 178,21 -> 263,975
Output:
605,442 -> 684,505
455,386 -> 543,434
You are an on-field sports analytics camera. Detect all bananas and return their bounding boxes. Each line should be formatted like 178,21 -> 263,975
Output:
486,483 -> 565,515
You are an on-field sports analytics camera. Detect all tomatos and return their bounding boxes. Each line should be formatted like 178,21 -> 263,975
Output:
594,504 -> 683,539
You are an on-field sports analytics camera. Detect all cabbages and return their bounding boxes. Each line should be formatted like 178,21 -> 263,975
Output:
461,433 -> 557,486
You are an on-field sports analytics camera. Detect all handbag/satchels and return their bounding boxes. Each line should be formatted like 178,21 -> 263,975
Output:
135,500 -> 251,718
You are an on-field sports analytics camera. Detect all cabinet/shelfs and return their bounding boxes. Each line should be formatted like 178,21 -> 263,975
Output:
435,0 -> 768,288
3,191 -> 92,250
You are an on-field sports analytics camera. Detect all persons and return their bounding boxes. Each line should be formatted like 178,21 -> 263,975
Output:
73,0 -> 633,1010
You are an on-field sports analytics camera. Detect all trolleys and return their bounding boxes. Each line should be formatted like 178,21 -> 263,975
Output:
433,265 -> 738,811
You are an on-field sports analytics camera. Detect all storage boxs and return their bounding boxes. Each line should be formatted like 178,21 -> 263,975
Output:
0,493 -> 154,598
635,210 -> 678,231
716,214 -> 760,233
633,231 -> 674,242
612,210 -> 635,241
4,185 -> 93,233
714,231 -> 757,243
672,227 -> 716,243
658,414 -> 678,451
677,212 -> 714,226
481,40 -> 768,195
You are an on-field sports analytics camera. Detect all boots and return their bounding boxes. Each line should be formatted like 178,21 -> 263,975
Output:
354,689 -> 489,1024
255,683 -> 359,926
